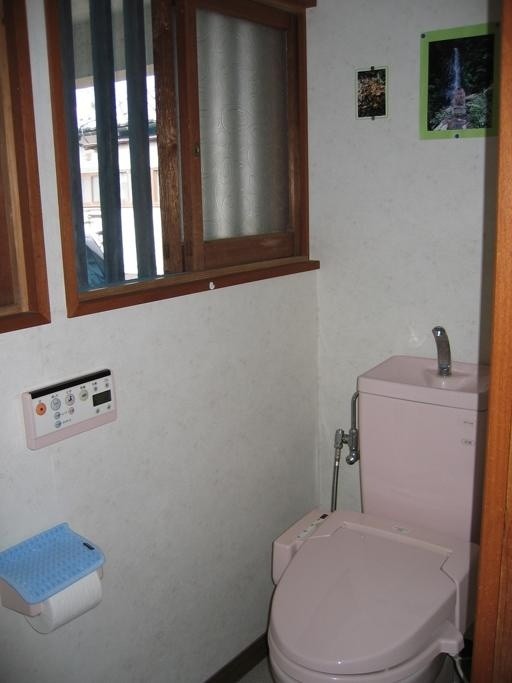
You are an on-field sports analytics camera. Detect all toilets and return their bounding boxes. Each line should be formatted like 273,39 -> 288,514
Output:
268,355 -> 490,683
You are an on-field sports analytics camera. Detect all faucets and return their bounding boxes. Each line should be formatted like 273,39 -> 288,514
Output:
431,326 -> 452,373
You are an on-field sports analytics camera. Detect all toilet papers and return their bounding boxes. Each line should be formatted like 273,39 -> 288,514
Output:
25,571 -> 101,635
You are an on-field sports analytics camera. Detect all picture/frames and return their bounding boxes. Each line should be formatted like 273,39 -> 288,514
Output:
355,21 -> 500,140
